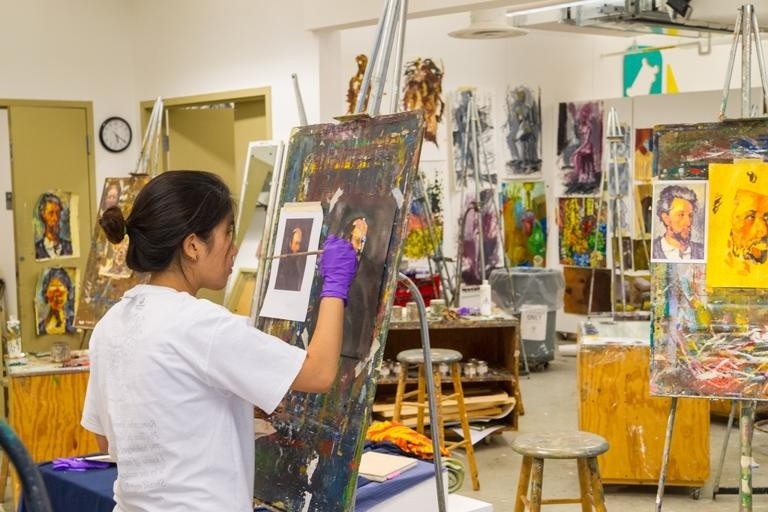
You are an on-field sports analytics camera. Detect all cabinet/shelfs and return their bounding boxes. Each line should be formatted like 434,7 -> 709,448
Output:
575,318 -> 711,490
0,354 -> 107,511
369,308 -> 526,444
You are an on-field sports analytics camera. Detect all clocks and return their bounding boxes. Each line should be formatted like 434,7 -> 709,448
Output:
98,115 -> 133,153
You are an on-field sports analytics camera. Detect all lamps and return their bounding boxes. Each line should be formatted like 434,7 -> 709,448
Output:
446,7 -> 532,42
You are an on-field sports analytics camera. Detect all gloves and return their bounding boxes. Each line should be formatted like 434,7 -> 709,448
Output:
317,233 -> 359,306
52,455 -> 110,470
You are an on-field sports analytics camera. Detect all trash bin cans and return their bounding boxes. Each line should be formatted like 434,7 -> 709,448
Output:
488,267 -> 565,367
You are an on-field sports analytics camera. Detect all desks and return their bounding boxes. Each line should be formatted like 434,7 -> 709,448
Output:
14,426 -> 490,510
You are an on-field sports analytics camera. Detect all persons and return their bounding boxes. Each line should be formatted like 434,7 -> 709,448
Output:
36,193 -> 71,258
343,217 -> 368,266
726,181 -> 768,275
38,268 -> 76,334
101,179 -> 124,216
110,234 -> 129,274
279,227 -> 301,291
652,184 -> 704,261
80,170 -> 360,512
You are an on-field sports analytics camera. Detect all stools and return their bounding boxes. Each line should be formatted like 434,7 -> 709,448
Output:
391,347 -> 482,493
502,428 -> 613,512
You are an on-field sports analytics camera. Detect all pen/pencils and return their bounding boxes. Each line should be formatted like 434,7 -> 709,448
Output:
387,463 -> 416,480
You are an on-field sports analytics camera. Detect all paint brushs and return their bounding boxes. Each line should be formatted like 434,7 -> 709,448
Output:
264,250 -> 324,259
